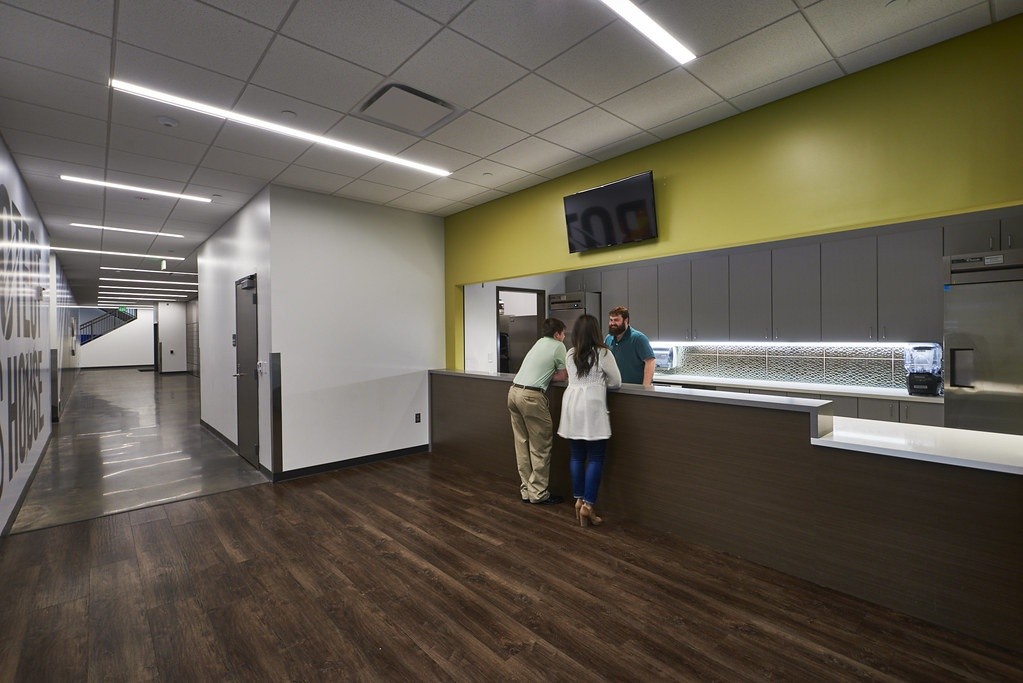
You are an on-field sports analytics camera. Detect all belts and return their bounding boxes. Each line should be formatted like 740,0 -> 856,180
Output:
511,382 -> 546,394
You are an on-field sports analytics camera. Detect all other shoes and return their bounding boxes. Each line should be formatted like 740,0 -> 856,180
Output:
540,494 -> 565,505
521,498 -> 531,503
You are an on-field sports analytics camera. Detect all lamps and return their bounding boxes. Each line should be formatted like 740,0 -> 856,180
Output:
161,259 -> 166,270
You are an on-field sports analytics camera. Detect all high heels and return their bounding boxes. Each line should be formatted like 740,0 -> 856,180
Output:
575,499 -> 584,519
580,506 -> 602,526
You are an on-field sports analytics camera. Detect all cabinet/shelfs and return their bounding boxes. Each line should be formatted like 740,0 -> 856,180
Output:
715,386 -> 945,427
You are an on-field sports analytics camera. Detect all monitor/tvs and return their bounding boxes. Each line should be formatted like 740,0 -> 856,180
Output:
562,169 -> 658,254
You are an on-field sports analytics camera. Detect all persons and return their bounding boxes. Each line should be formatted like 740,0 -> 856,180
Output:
556,315 -> 622,528
507,318 -> 568,504
604,304 -> 656,385
